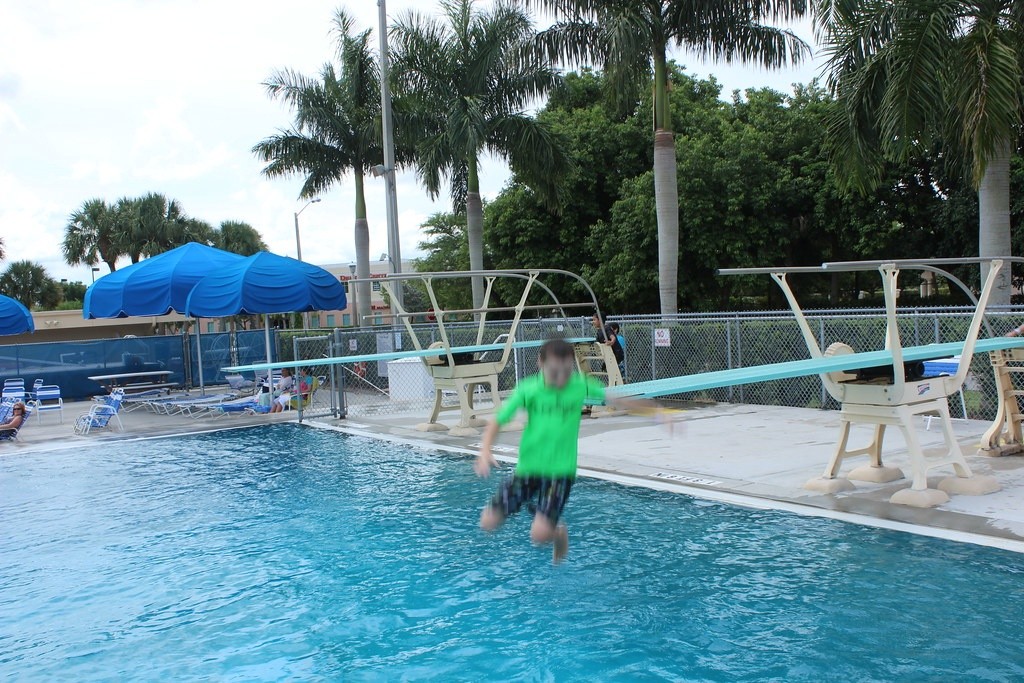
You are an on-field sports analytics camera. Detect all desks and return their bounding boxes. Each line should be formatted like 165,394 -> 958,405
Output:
87,371 -> 174,392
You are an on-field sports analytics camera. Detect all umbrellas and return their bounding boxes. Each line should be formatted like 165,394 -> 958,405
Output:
83,243 -> 347,392
0,294 -> 34,336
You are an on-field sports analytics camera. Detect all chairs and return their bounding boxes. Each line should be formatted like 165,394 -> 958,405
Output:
0,360 -> 327,445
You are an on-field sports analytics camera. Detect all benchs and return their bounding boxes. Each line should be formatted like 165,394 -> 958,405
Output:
120,382 -> 180,395
100,380 -> 166,393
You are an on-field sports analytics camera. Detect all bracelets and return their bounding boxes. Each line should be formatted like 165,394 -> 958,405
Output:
1014,328 -> 1021,335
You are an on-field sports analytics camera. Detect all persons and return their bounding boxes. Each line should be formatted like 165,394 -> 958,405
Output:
582,313 -> 626,416
0,403 -> 25,437
523,439 -> 525,440
476,340 -> 661,562
270,366 -> 313,413
